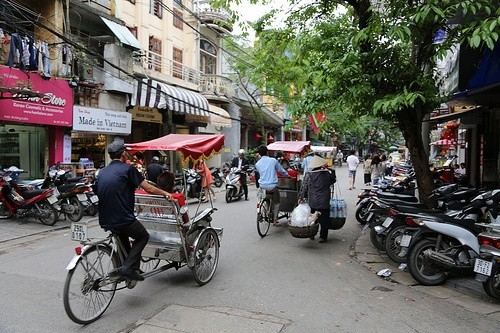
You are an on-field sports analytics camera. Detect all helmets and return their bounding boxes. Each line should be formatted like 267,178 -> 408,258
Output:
238,149 -> 246,155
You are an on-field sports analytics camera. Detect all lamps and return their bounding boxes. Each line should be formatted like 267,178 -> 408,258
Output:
55,77 -> 79,88
40,72 -> 52,80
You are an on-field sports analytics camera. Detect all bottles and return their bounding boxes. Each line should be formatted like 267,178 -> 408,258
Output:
329,198 -> 347,218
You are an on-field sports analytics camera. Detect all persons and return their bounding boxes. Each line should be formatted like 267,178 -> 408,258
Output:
74,145 -> 466,243
97,141 -> 176,281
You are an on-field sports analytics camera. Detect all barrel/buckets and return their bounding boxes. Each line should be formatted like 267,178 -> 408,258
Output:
277,170 -> 298,184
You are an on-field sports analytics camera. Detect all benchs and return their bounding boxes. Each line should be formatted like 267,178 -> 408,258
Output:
133,194 -> 218,244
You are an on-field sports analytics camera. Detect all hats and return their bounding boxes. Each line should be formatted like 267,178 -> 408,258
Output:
107,142 -> 125,155
151,156 -> 160,161
307,155 -> 328,169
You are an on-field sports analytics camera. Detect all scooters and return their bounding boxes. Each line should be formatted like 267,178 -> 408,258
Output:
0,160 -> 101,226
353,160 -> 500,304
222,161 -> 253,204
161,159 -> 225,203
333,156 -> 342,168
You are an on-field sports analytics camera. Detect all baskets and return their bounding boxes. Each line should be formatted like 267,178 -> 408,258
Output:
329,217 -> 346,230
287,222 -> 319,238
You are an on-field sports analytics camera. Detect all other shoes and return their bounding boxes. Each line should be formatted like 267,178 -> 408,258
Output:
319,238 -> 326,242
273,220 -> 279,226
120,267 -> 145,281
245,198 -> 249,201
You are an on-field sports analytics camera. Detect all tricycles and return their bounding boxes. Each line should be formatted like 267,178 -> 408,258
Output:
61,132 -> 225,326
253,140 -> 312,238
310,145 -> 337,168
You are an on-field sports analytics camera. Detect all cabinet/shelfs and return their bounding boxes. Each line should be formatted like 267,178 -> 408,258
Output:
0,131 -> 30,178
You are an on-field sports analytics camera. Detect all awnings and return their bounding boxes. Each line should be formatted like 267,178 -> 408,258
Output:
101,18 -> 144,50
126,78 -> 232,129
